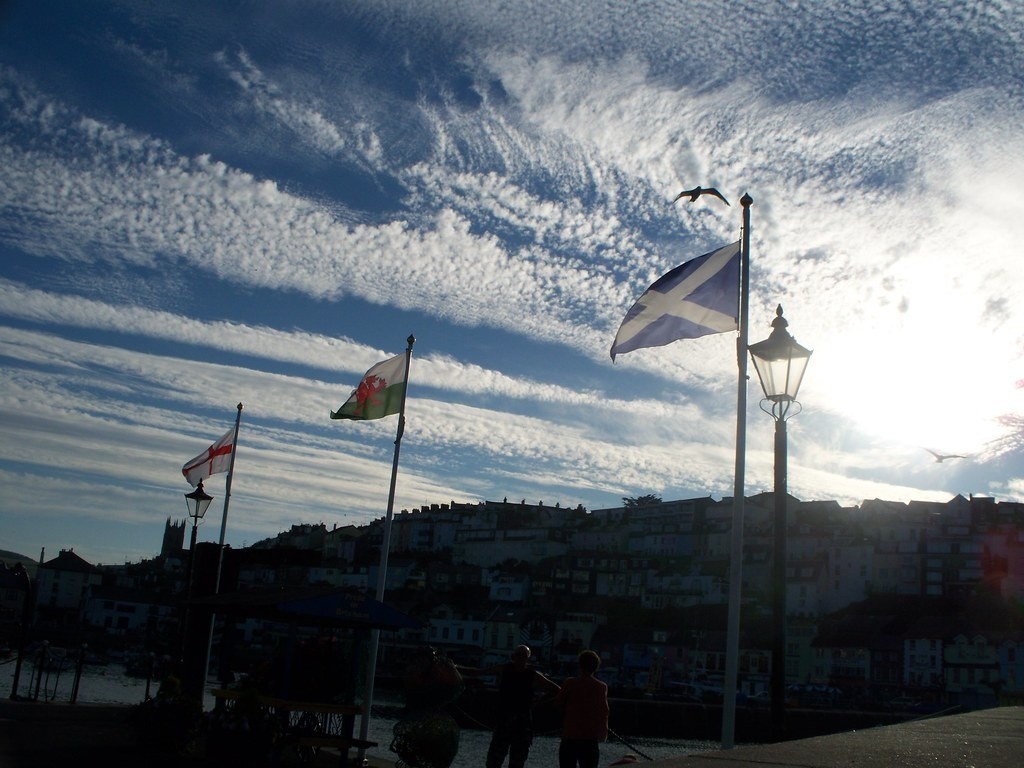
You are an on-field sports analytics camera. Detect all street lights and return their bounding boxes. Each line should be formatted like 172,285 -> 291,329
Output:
744,302 -> 812,743
163,478 -> 215,705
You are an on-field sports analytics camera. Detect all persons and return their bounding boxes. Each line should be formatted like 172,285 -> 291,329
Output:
405,644 -> 463,712
554,650 -> 609,768
487,645 -> 544,768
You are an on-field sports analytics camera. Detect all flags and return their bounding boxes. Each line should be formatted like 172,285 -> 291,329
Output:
610,240 -> 739,362
330,352 -> 406,420
182,428 -> 232,487
